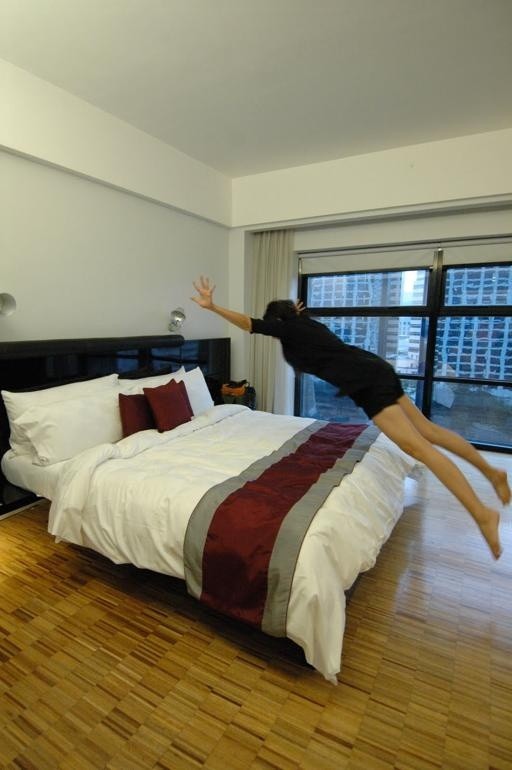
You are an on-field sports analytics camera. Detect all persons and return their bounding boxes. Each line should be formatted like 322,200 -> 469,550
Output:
189,274 -> 511,560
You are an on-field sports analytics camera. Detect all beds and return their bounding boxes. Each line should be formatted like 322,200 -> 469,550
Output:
0,403 -> 426,686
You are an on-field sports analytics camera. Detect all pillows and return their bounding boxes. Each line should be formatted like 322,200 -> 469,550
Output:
1,365 -> 215,469
